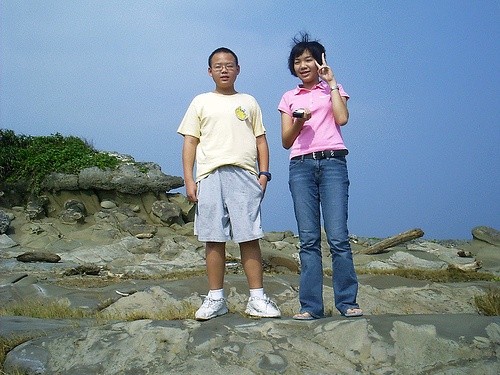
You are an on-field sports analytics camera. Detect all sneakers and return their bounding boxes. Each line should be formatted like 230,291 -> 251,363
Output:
194,294 -> 229,319
245,293 -> 281,317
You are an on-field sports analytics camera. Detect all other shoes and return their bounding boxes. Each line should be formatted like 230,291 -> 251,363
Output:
293,312 -> 315,319
345,308 -> 364,316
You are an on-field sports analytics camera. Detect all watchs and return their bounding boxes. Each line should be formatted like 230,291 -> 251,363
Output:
259,171 -> 271,181
330,85 -> 340,91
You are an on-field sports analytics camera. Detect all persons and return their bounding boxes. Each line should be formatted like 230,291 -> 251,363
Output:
278,31 -> 365,319
175,47 -> 281,320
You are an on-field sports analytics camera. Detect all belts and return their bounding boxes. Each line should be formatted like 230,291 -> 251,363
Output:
291,149 -> 348,159
